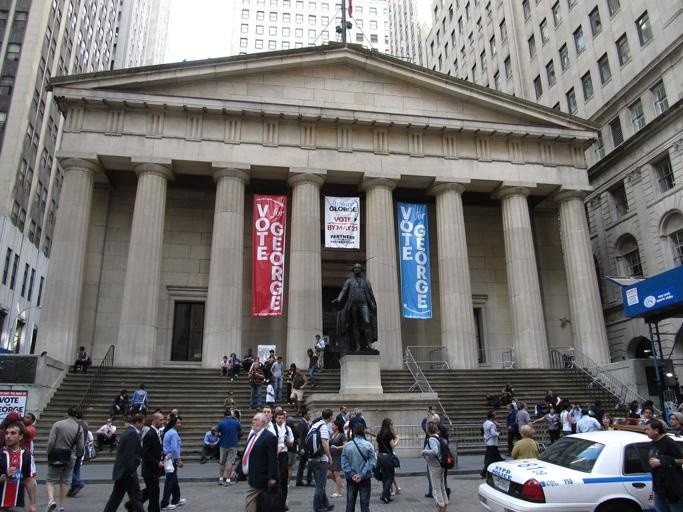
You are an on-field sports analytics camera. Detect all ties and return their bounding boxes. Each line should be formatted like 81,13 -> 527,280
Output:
241,433 -> 258,464
158,431 -> 162,445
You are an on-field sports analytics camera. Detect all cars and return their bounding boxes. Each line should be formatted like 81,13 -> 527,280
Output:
472,424 -> 670,512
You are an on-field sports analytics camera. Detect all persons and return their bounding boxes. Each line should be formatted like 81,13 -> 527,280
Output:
0,408 -> 94,512
202,404 -> 400,512
105,408 -> 187,512
71,345 -> 90,374
218,332 -> 328,417
480,384 -> 683,512
420,411 -> 449,512
96,384 -> 148,454
331,263 -> 380,355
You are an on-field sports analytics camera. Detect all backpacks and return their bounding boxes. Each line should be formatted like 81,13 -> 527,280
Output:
304,423 -> 329,458
428,435 -> 455,468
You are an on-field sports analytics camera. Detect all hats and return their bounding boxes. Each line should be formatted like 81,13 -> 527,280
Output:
668,412 -> 683,424
6,413 -> 18,422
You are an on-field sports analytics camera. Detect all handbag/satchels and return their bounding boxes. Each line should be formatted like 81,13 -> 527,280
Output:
48,449 -> 72,466
256,485 -> 287,512
391,454 -> 400,468
85,445 -> 96,459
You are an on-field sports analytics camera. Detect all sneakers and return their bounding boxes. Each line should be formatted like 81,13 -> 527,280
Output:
218,477 -> 234,486
176,498 -> 187,506
296,481 -> 346,512
161,504 -> 176,511
46,499 -> 57,512
57,507 -> 64,512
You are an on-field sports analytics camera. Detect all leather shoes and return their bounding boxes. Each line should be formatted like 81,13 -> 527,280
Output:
69,486 -> 82,497
424,493 -> 433,497
380,487 -> 401,503
